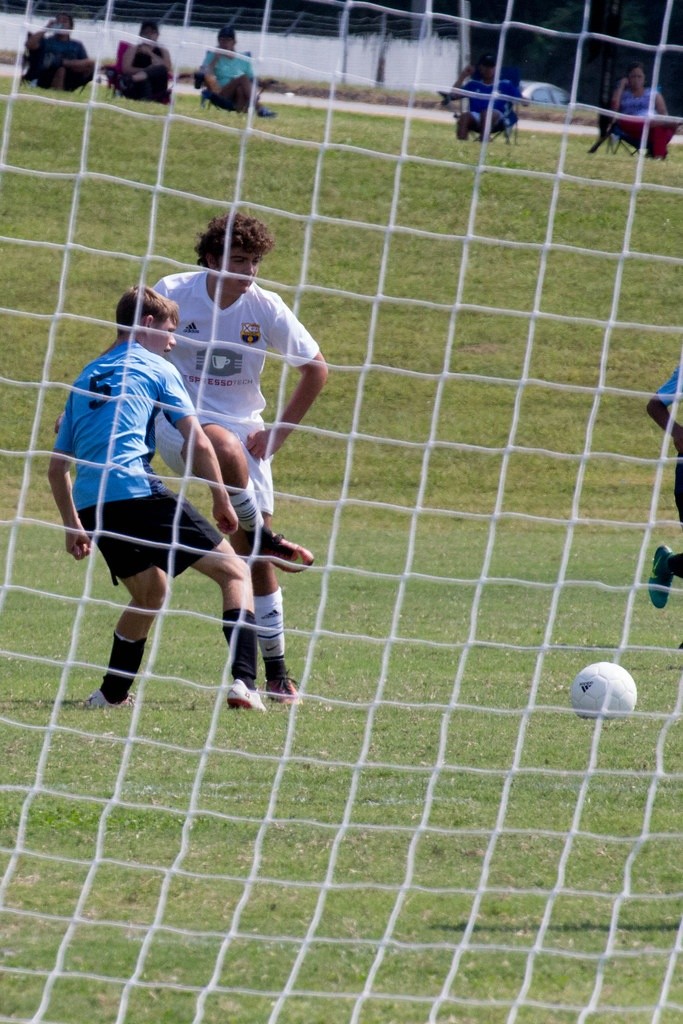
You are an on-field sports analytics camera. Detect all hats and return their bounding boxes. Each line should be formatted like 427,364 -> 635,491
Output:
481,54 -> 494,68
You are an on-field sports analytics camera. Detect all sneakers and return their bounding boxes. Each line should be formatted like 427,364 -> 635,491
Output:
226,679 -> 265,711
267,667 -> 303,706
86,689 -> 136,708
249,531 -> 314,572
649,544 -> 673,608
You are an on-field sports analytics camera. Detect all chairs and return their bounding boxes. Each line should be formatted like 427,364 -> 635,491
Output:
439,62 -> 521,148
102,41 -> 174,102
195,51 -> 277,117
26,30 -> 92,91
598,103 -> 676,156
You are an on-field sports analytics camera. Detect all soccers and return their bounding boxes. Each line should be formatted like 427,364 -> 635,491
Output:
569,660 -> 639,721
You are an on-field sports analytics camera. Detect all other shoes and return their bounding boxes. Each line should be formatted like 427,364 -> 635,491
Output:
259,109 -> 276,117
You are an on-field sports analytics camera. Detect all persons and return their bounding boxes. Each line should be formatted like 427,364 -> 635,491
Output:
48,286 -> 266,710
647,365 -> 683,608
21,13 -> 96,91
152,213 -> 328,705
122,22 -> 172,103
204,28 -> 277,117
612,64 -> 678,161
452,56 -> 529,142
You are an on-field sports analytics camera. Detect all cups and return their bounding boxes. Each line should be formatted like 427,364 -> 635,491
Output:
49,22 -> 62,30
